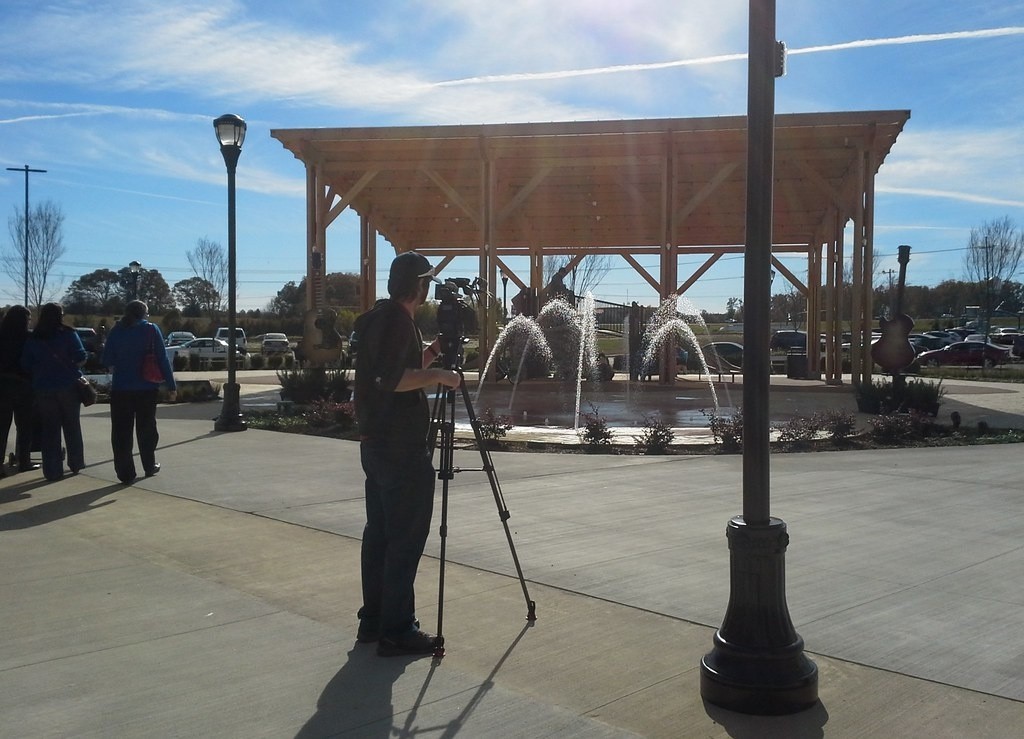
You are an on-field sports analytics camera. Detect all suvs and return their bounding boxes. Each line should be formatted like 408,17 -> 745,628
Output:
214,328 -> 249,356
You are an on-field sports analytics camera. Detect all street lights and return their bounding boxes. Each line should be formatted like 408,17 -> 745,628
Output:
129,259 -> 142,302
5,165 -> 48,309
213,113 -> 248,433
499,268 -> 509,326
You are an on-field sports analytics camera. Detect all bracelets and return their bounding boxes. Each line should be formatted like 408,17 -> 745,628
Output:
428,344 -> 439,358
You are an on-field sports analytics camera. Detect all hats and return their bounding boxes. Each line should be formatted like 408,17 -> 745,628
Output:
389,251 -> 442,286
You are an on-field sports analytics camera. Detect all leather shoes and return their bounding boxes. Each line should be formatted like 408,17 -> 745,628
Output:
146,462 -> 161,477
122,480 -> 132,485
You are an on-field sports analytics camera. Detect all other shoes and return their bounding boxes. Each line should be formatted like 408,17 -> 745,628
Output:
28,462 -> 41,471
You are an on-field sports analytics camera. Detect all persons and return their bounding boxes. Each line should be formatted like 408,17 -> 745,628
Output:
0,303 -> 87,481
357,250 -> 462,655
97,300 -> 178,483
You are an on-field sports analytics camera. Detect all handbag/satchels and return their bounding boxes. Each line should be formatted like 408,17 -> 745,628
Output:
139,323 -> 167,383
77,375 -> 97,407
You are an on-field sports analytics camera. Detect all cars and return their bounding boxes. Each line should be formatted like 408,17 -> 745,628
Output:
348,330 -> 358,351
687,342 -> 744,372
163,332 -> 196,349
163,338 -> 243,364
917,340 -> 1013,371
260,333 -> 289,356
720,323 -> 743,334
771,310 -> 1024,361
74,328 -> 98,346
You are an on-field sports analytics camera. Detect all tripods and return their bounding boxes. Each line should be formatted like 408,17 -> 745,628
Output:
424,354 -> 539,656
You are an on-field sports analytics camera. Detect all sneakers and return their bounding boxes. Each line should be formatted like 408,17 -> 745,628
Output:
356,618 -> 420,640
377,631 -> 444,657
